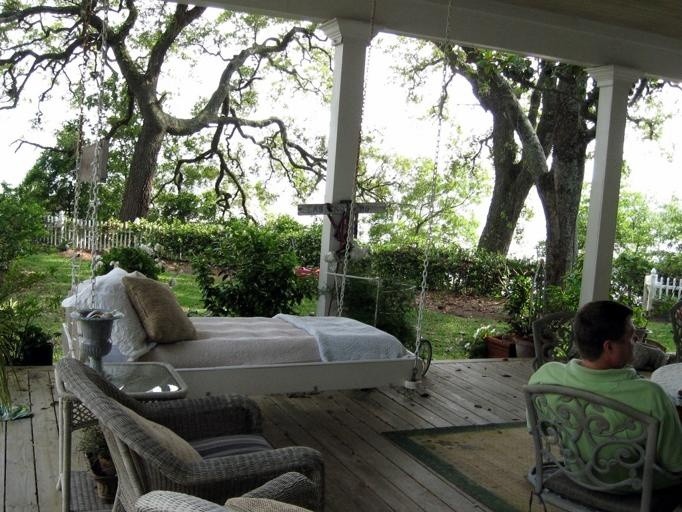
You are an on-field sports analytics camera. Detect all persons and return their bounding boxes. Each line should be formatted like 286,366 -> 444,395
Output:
524,301 -> 682,512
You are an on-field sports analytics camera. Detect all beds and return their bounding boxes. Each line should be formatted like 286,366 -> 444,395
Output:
58,315 -> 420,400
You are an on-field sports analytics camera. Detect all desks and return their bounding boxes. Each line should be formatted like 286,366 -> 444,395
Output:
53,358 -> 189,512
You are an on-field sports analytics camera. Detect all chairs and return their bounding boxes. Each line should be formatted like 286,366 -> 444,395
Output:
520,383 -> 661,512
60,357 -> 327,512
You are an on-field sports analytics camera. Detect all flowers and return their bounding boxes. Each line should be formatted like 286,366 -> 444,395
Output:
504,271 -> 544,337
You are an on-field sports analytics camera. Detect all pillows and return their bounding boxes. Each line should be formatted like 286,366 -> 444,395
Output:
59,263 -> 197,363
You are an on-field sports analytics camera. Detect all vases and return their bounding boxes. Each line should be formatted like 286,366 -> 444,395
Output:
486,334 -> 535,359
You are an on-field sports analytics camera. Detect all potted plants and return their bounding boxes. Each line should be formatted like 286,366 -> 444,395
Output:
17,322 -> 62,367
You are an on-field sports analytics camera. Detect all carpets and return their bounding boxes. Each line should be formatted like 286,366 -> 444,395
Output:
380,421 -> 565,511
